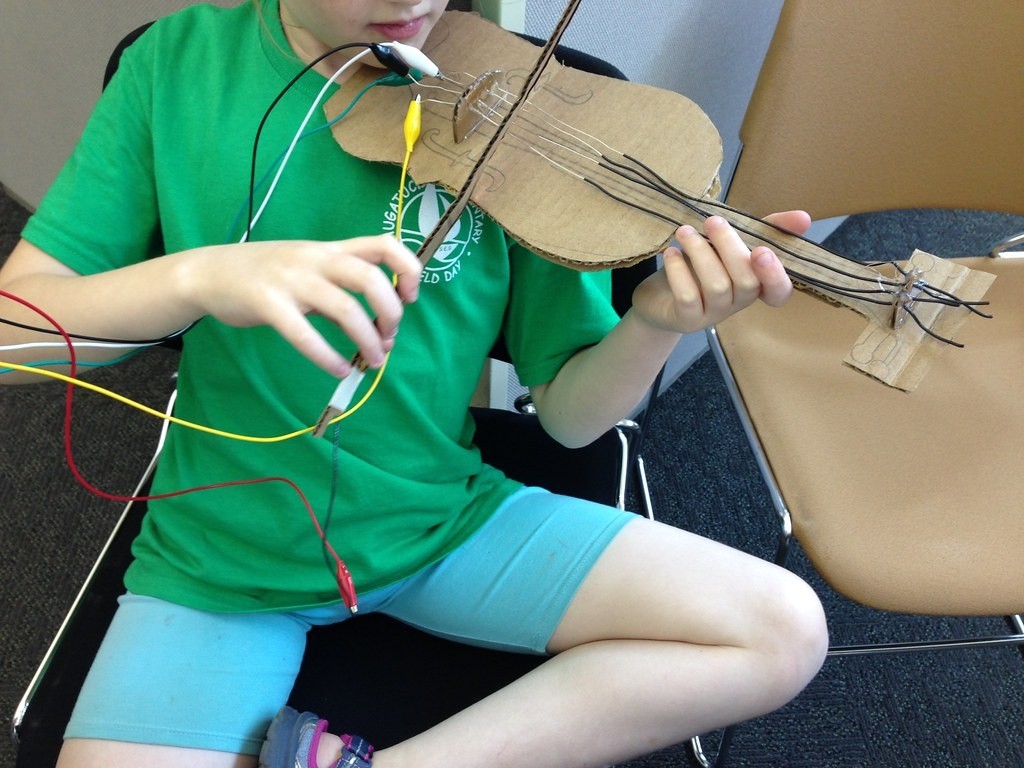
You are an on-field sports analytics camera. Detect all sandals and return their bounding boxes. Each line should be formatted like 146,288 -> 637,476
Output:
258,706 -> 373,768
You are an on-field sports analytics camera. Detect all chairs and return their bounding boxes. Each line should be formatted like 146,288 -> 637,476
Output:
11,19 -> 629,768
632,0 -> 1024,768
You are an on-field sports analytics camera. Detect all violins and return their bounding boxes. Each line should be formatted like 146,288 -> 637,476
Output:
320,4 -> 1000,399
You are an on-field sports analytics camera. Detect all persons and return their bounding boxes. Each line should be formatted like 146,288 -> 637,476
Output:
0,0 -> 829,768
